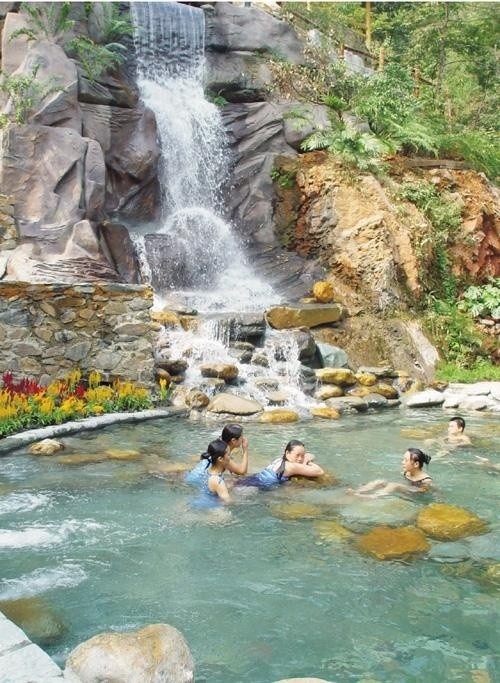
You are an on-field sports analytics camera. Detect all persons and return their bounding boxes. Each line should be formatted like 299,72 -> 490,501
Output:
345,446 -> 435,501
181,421 -> 250,496
429,415 -> 475,462
173,438 -> 236,524
232,438 -> 327,505
